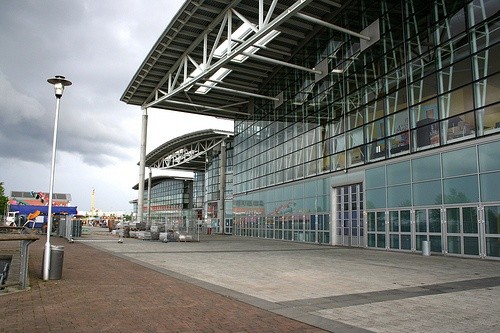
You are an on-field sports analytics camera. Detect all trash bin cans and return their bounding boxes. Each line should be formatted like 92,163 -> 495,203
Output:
124,225 -> 130,239
421,240 -> 432,257
41,242 -> 65,280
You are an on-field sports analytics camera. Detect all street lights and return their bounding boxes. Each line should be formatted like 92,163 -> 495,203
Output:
40,74 -> 72,283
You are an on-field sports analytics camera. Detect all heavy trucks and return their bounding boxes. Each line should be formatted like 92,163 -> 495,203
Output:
5,204 -> 77,228
